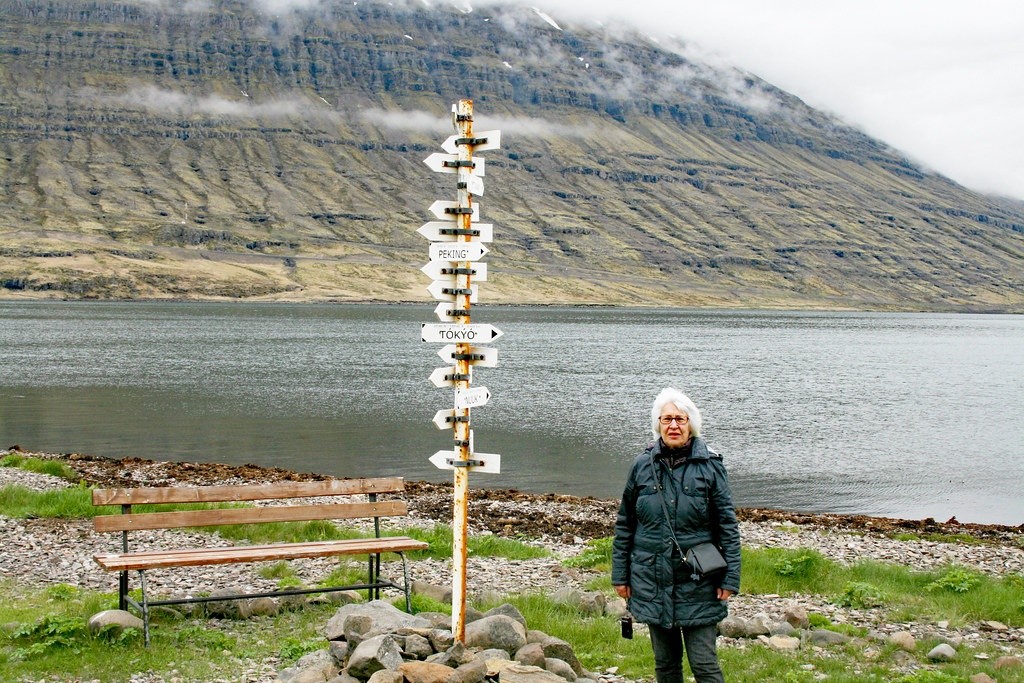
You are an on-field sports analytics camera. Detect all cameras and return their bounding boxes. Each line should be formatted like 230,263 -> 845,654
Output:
621,617 -> 633,639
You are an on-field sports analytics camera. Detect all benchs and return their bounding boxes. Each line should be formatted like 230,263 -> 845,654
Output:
91,476 -> 428,648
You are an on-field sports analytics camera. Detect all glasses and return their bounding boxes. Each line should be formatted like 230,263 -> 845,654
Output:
657,414 -> 690,426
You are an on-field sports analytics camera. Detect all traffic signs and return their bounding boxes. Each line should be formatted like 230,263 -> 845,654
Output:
427,242 -> 490,261
419,322 -> 503,343
457,386 -> 492,409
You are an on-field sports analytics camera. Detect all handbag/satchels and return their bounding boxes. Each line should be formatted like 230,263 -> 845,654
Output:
681,542 -> 728,578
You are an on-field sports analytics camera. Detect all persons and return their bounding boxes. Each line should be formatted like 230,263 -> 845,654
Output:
610,388 -> 742,683
